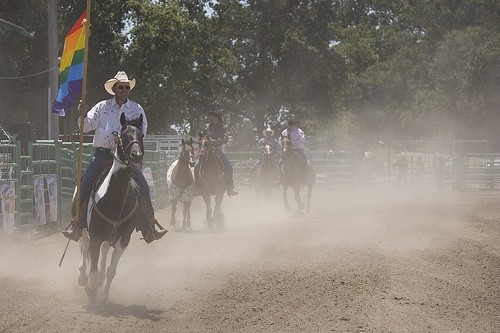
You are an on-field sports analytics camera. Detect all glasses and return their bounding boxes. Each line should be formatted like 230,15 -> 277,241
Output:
114,85 -> 130,91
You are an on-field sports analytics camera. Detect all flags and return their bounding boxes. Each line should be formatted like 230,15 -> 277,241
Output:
52,10 -> 87,117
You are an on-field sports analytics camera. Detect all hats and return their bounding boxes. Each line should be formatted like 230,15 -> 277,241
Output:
104,70 -> 136,96
263,127 -> 274,136
209,110 -> 222,121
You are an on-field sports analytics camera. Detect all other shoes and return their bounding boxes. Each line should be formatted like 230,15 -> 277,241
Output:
62,227 -> 82,242
228,185 -> 234,195
143,226 -> 168,244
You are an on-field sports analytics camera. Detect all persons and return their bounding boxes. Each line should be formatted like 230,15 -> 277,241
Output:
194,111 -> 237,197
63,71 -> 167,242
394,152 -> 408,185
251,127 -> 281,175
277,120 -> 307,186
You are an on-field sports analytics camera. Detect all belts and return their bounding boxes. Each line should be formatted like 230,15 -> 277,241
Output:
97,147 -> 111,152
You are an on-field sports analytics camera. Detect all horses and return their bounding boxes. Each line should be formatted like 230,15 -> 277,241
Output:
70,112 -> 145,304
280,132 -> 315,214
255,145 -> 277,184
193,136 -> 225,231
165,138 -> 195,232
